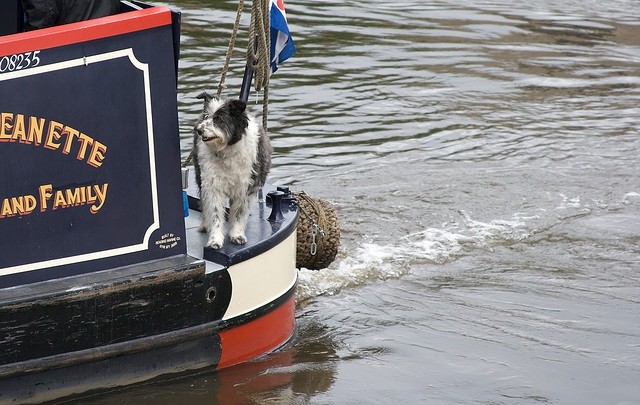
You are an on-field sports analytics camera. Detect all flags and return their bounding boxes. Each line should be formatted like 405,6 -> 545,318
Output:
269,0 -> 296,80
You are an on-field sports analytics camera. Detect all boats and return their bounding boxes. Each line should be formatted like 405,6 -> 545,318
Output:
0,0 -> 340,405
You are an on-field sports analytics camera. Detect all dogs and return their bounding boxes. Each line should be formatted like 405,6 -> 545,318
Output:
191,92 -> 274,250
